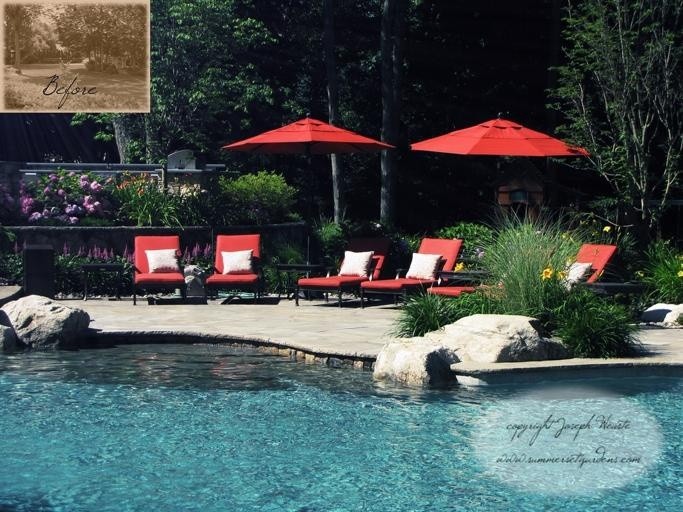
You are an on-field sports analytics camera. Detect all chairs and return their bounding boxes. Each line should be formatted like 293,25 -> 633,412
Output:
133,235 -> 187,303
206,235 -> 262,304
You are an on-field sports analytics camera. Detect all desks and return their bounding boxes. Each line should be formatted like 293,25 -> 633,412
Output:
81,263 -> 125,300
276,263 -> 327,303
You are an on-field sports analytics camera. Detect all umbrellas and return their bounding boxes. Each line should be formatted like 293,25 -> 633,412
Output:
409,112 -> 591,216
222,111 -> 396,279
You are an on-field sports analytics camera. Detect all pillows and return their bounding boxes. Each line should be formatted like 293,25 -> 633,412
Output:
144,248 -> 180,275
220,248 -> 254,277
